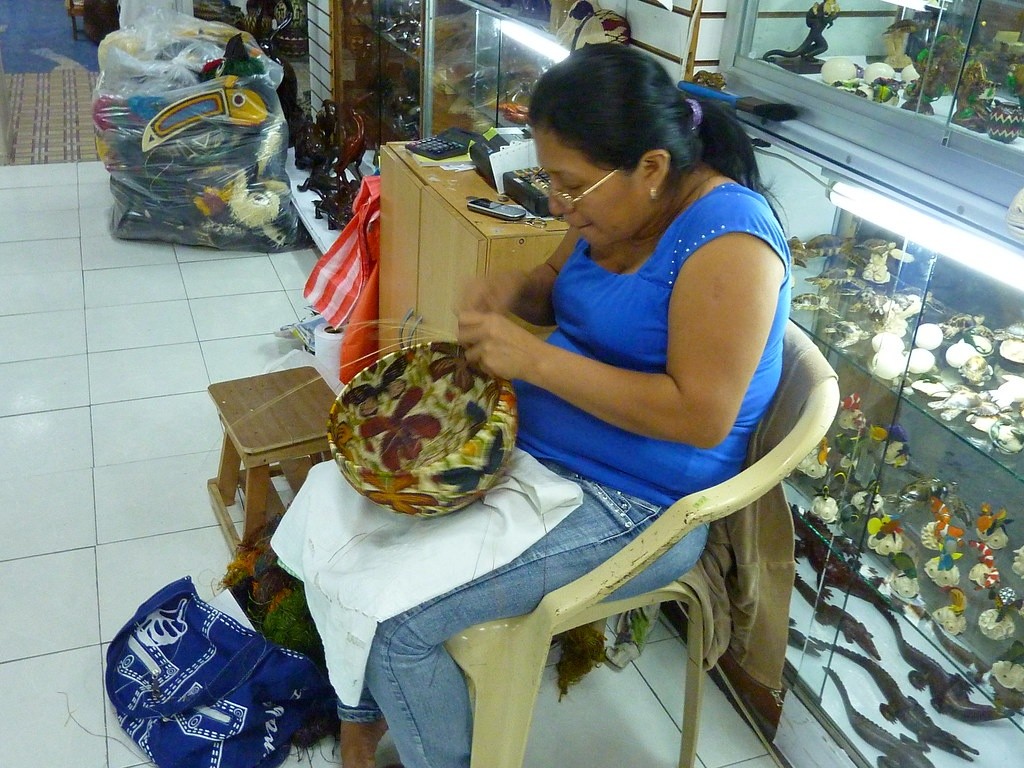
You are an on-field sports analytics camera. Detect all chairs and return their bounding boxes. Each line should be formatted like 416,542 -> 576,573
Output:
441,319 -> 843,768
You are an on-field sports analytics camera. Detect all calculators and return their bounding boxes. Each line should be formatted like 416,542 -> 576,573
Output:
404,127 -> 482,161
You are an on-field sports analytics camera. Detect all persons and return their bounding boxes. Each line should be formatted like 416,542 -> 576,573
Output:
336,42 -> 792,768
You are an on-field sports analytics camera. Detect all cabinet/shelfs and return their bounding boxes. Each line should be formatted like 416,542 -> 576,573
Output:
377,143 -> 568,357
719,0 -> 1024,768
330,0 -> 570,165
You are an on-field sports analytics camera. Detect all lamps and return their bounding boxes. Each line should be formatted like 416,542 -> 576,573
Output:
819,168 -> 1024,291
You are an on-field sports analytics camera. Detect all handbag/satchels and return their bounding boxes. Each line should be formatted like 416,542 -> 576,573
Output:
105,576 -> 323,768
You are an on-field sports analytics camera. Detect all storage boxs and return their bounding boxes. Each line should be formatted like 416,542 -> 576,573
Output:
626,0 -> 904,89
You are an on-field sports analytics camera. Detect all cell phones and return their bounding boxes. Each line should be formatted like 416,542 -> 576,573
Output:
466,196 -> 527,222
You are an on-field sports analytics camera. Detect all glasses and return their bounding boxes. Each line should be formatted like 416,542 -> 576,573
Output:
534,166 -> 618,213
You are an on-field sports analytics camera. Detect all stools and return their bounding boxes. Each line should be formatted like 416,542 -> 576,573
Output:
205,365 -> 341,561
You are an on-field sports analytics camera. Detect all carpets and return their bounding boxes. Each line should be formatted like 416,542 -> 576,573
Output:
0,0 -> 312,167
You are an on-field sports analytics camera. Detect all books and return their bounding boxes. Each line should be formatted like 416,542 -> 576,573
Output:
406,148 -> 475,166
